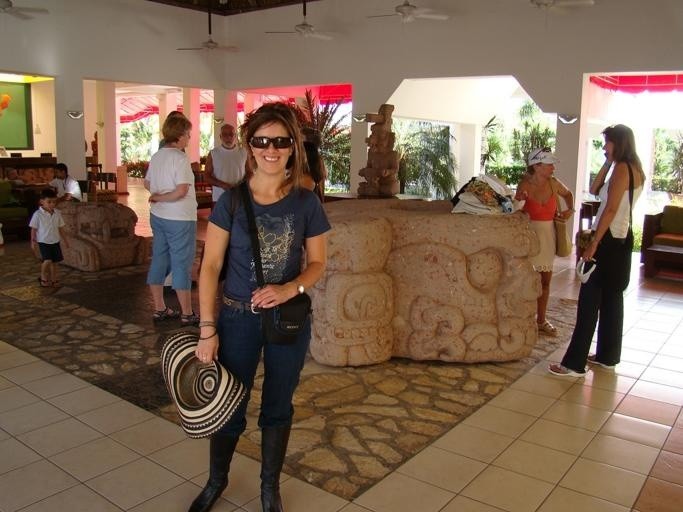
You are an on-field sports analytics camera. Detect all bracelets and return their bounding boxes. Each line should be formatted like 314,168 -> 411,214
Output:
594,239 -> 600,243
567,206 -> 576,212
603,161 -> 611,171
198,321 -> 215,328
198,331 -> 216,341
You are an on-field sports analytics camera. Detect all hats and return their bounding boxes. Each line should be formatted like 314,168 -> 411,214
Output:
161,331 -> 247,438
528,147 -> 559,166
576,258 -> 596,284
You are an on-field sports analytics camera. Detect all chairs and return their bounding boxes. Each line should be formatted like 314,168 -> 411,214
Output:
0,165 -> 118,241
576,202 -> 601,267
641,212 -> 683,264
195,172 -> 214,216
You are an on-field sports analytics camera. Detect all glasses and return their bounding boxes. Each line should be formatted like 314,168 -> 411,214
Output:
250,136 -> 293,148
532,147 -> 551,158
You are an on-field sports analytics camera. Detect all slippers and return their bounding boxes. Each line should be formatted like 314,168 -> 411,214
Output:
587,355 -> 616,368
548,363 -> 585,377
39,277 -> 61,288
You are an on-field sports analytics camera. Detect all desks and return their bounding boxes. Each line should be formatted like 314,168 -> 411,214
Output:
645,244 -> 683,278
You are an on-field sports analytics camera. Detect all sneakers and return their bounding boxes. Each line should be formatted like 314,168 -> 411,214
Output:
538,320 -> 556,332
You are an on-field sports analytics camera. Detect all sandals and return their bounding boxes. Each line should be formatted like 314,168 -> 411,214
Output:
153,307 -> 200,325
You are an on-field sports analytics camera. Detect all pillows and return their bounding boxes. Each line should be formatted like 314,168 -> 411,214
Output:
660,205 -> 683,235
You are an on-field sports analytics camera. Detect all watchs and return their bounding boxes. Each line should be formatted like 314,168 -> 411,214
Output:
292,279 -> 304,295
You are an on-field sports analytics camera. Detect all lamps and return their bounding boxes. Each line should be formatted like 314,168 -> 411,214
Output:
353,114 -> 366,123
68,111 -> 83,120
96,121 -> 104,128
214,118 -> 224,124
34,75 -> 41,135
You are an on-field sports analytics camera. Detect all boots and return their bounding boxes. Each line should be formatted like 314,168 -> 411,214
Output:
188,422 -> 246,512
260,426 -> 291,512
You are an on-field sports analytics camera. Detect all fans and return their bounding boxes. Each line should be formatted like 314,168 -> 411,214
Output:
365,0 -> 450,24
175,0 -> 240,54
263,0 -> 337,41
0,0 -> 50,21
528,0 -> 595,13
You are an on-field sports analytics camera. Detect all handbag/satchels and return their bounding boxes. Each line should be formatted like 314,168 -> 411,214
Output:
556,218 -> 572,256
260,300 -> 308,344
588,228 -> 633,292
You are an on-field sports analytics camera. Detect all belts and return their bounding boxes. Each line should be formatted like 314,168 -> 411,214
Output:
223,296 -> 264,314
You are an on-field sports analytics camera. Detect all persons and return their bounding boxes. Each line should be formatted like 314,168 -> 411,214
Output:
188,112 -> 331,510
27,189 -> 69,289
202,123 -> 250,215
254,102 -> 315,192
514,146 -> 573,334
143,116 -> 200,325
303,141 -> 327,203
548,123 -> 644,378
159,110 -> 186,152
43,163 -> 82,202
